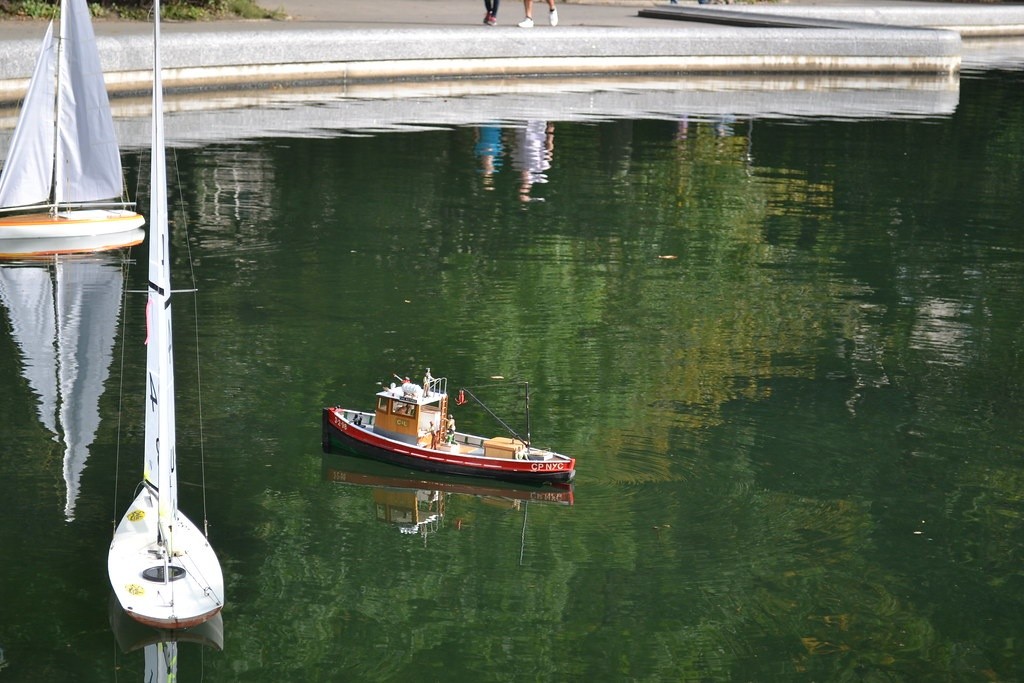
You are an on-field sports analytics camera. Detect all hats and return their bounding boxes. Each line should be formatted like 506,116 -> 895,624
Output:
430,421 -> 434,426
448,414 -> 453,419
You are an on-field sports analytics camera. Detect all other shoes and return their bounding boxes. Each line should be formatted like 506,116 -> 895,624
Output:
483,11 -> 497,26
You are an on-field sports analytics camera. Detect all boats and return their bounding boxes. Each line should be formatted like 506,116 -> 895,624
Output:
328,466 -> 575,569
322,366 -> 577,485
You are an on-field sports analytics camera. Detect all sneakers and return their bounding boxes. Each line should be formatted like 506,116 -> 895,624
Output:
518,18 -> 533,27
549,8 -> 558,27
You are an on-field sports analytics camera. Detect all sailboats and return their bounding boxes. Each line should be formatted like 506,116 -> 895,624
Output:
106,589 -> 224,683
0,252 -> 133,523
107,0 -> 225,628
1,1 -> 146,259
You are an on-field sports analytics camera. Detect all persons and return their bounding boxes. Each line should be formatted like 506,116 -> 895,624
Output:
422,367 -> 437,398
441,413 -> 459,446
481,1 -> 559,27
421,421 -> 437,450
